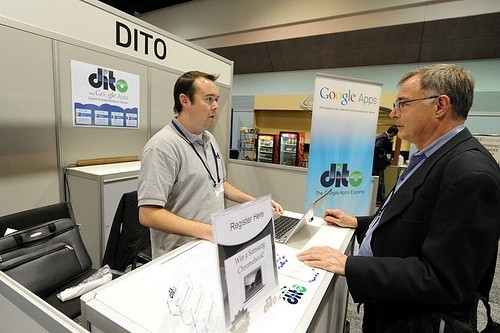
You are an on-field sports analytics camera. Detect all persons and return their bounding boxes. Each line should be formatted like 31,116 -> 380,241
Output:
136,71 -> 284,260
296,64 -> 500,333
372,125 -> 399,205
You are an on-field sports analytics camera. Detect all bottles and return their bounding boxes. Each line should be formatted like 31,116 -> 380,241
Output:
286,138 -> 297,145
259,139 -> 273,163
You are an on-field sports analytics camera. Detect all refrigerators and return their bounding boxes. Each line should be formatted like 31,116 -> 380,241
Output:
279,130 -> 306,167
256,133 -> 279,164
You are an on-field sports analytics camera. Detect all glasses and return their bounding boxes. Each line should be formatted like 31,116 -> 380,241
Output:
393,96 -> 439,110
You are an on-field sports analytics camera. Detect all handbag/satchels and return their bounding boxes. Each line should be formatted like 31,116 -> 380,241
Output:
419,146 -> 500,333
0,218 -> 93,299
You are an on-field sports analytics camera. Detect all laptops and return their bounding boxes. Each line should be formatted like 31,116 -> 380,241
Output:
274,189 -> 331,244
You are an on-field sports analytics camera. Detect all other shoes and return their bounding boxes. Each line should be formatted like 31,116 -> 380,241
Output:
377,199 -> 386,202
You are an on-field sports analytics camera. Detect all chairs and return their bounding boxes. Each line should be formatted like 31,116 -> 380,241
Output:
100,191 -> 152,270
